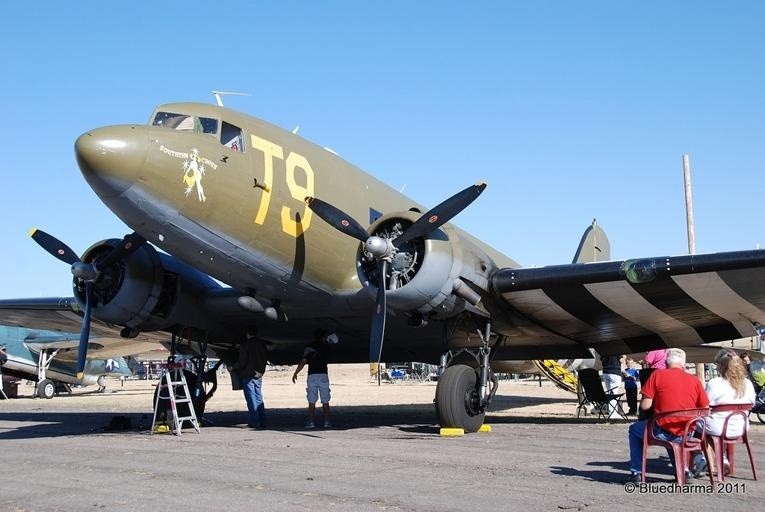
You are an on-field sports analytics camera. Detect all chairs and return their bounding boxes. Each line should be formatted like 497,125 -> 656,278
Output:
575,367 -> 632,423
641,407 -> 718,493
696,402 -> 758,483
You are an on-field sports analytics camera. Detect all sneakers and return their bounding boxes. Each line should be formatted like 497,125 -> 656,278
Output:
623,474 -> 642,487
723,456 -> 731,477
304,420 -> 315,428
323,421 -> 332,428
674,455 -> 706,483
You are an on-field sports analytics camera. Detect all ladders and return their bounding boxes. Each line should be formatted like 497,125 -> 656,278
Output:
151,363 -> 200,437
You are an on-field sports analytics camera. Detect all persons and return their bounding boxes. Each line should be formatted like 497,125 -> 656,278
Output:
226,324 -> 270,430
291,327 -> 339,429
624,347 -> 765,488
566,354 -> 625,415
